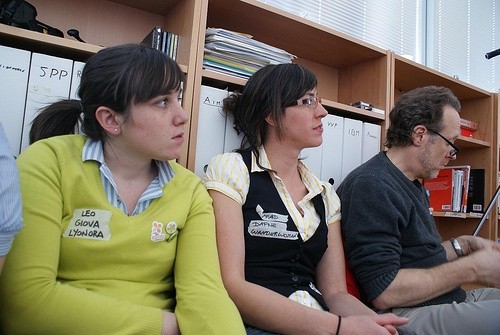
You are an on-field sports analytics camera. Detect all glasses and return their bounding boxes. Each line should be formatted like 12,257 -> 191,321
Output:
411,125 -> 459,157
282,93 -> 322,111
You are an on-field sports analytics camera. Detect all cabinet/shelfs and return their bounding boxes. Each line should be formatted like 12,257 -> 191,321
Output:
188,0 -> 390,181
0,0 -> 201,157
392,51 -> 500,292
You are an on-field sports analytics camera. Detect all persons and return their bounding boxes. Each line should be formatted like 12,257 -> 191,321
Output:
0,44 -> 249,335
335,85 -> 500,335
202,64 -> 409,335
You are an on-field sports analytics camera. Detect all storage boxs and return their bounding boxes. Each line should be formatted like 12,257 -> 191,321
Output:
0,46 -> 87,158
197,85 -> 382,194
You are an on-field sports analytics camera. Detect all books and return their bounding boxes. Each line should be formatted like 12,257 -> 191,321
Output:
138,25 -> 178,62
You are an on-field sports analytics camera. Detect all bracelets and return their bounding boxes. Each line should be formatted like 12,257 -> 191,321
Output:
450,237 -> 463,257
336,315 -> 341,335
